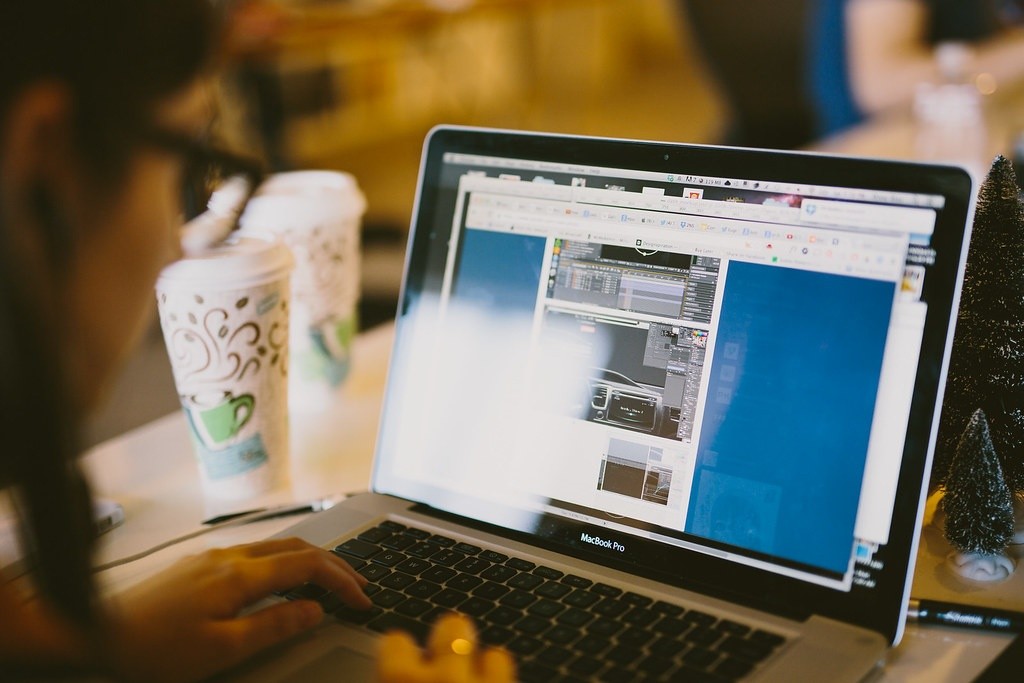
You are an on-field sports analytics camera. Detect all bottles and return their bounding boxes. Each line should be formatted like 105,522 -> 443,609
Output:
913,44 -> 980,206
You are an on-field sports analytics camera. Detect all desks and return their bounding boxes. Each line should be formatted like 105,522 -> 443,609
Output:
0,322 -> 1024,683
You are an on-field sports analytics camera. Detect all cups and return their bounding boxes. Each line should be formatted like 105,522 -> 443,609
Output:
155,241 -> 293,514
246,170 -> 368,405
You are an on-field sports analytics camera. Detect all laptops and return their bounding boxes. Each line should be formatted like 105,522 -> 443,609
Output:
207,128 -> 979,683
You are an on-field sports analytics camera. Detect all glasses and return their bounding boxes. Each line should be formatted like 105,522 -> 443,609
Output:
64,85 -> 266,255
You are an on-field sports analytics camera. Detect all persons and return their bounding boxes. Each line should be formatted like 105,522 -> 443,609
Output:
0,1 -> 515,683
808,0 -> 1024,139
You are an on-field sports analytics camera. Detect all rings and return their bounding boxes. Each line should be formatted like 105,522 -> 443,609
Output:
428,639 -> 476,662
